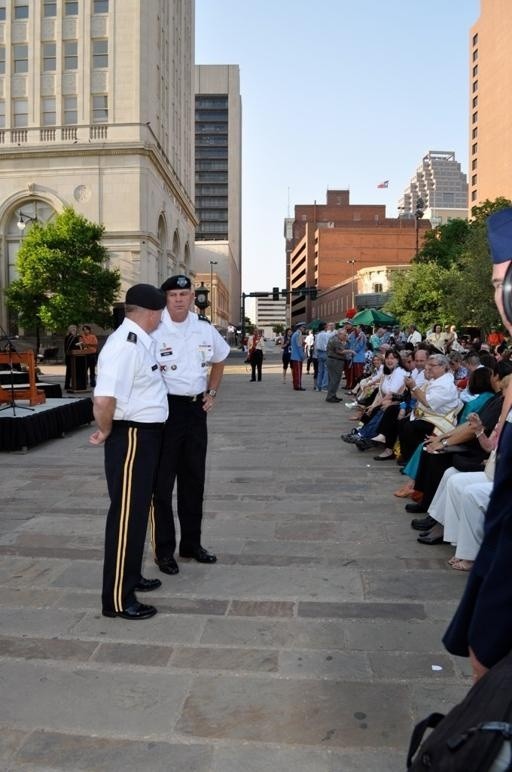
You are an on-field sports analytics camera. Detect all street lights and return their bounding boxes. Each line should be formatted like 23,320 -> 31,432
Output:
347,259 -> 358,309
415,210 -> 423,255
209,261 -> 218,323
17,212 -> 36,230
195,282 -> 209,317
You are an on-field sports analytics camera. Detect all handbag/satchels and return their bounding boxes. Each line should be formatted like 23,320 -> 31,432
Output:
409,652 -> 512,770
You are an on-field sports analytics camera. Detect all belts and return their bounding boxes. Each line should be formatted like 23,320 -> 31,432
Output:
167,392 -> 204,402
113,419 -> 167,429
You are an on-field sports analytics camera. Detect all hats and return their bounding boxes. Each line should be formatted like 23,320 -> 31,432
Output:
160,274 -> 191,291
295,321 -> 306,327
126,283 -> 166,310
345,319 -> 353,325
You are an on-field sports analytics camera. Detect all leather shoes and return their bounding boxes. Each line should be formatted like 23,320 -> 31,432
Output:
154,553 -> 179,575
132,575 -> 161,592
394,484 -> 474,570
102,601 -> 157,620
180,548 -> 216,563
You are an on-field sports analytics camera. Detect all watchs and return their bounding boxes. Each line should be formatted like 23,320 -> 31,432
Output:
206,388 -> 218,398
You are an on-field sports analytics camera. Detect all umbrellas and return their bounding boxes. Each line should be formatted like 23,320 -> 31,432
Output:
350,308 -> 401,330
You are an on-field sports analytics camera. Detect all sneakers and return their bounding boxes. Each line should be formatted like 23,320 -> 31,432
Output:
341,401 -> 396,460
294,386 -> 357,402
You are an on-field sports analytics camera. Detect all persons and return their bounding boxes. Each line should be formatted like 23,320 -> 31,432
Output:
63,326 -> 79,389
81,325 -> 96,386
89,283 -> 170,623
244,327 -> 267,383
150,274 -> 230,575
490,260 -> 511,333
278,319 -> 511,685
